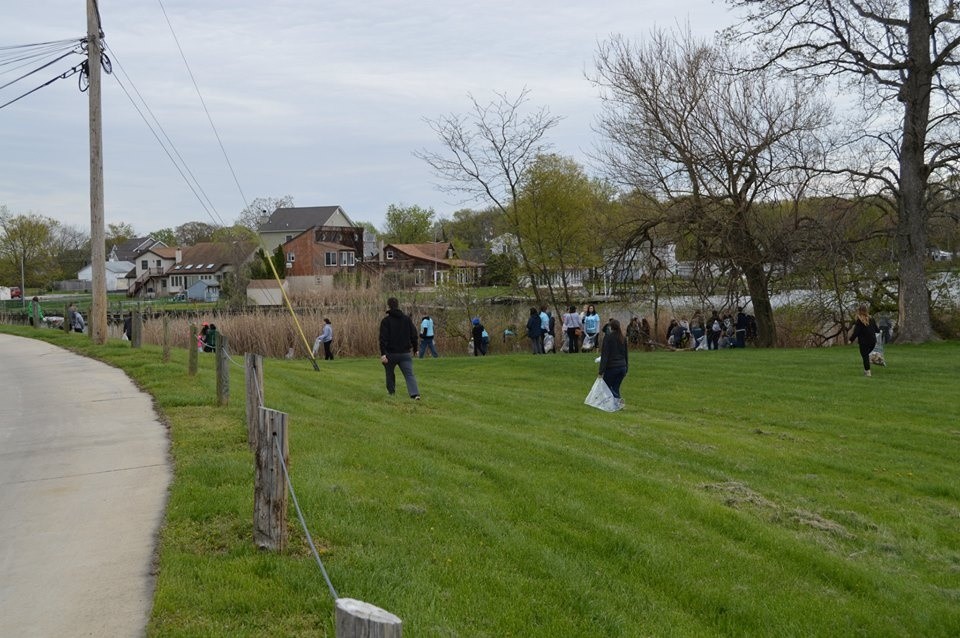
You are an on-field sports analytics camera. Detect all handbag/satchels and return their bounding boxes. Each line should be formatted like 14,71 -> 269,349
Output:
481,330 -> 489,344
121,333 -> 128,340
575,329 -> 581,336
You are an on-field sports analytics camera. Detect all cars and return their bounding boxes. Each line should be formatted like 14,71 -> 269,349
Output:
9,287 -> 20,297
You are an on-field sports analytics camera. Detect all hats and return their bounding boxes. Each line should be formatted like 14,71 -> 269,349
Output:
472,319 -> 480,326
856,304 -> 867,311
570,306 -> 577,310
201,321 -> 209,326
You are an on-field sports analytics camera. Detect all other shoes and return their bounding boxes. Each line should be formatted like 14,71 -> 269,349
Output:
410,395 -> 420,400
864,370 -> 871,377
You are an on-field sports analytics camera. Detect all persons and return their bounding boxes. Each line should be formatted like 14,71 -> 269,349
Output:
879,311 -> 891,343
665,306 -> 756,350
472,318 -> 489,356
379,298 -> 421,400
418,310 -> 439,359
562,304 -> 601,353
319,318 -> 334,360
526,305 -> 557,354
197,322 -> 219,352
627,317 -> 651,351
848,305 -> 880,376
123,311 -> 132,341
68,306 -> 84,333
598,318 -> 628,399
28,296 -> 46,326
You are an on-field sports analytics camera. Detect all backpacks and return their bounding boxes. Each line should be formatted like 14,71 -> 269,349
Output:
711,318 -> 721,332
